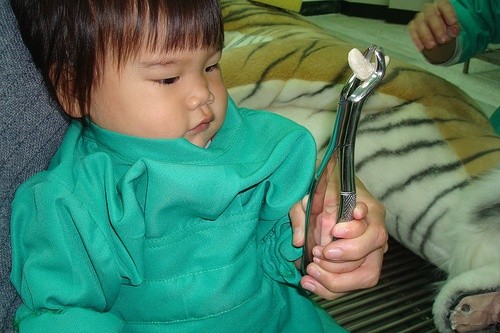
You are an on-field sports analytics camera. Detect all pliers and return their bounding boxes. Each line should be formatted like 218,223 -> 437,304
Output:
303,43 -> 386,282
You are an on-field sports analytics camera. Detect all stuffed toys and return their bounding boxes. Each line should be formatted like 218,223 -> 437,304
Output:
219,0 -> 500,333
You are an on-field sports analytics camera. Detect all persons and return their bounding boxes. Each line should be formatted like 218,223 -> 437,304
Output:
10,0 -> 367,333
0,0 -> 389,333
406,0 -> 500,136
